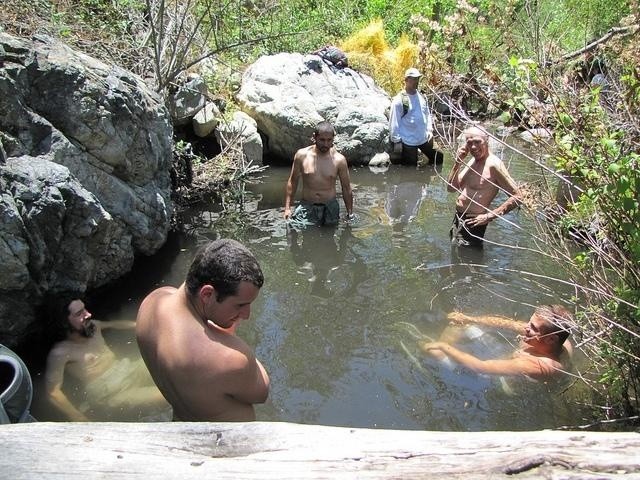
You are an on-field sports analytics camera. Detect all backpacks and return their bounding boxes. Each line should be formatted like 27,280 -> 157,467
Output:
313,44 -> 348,67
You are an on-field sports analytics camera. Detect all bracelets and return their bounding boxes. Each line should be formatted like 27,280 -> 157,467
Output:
485,212 -> 493,223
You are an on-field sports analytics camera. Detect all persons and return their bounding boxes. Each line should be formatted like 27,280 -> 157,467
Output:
40,292 -> 170,420
447,127 -> 528,249
382,164 -> 435,250
416,303 -> 575,399
385,65 -> 445,167
133,237 -> 272,420
283,221 -> 355,301
282,121 -> 354,229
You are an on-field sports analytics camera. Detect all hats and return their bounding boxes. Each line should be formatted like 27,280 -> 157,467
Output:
405,68 -> 423,78
